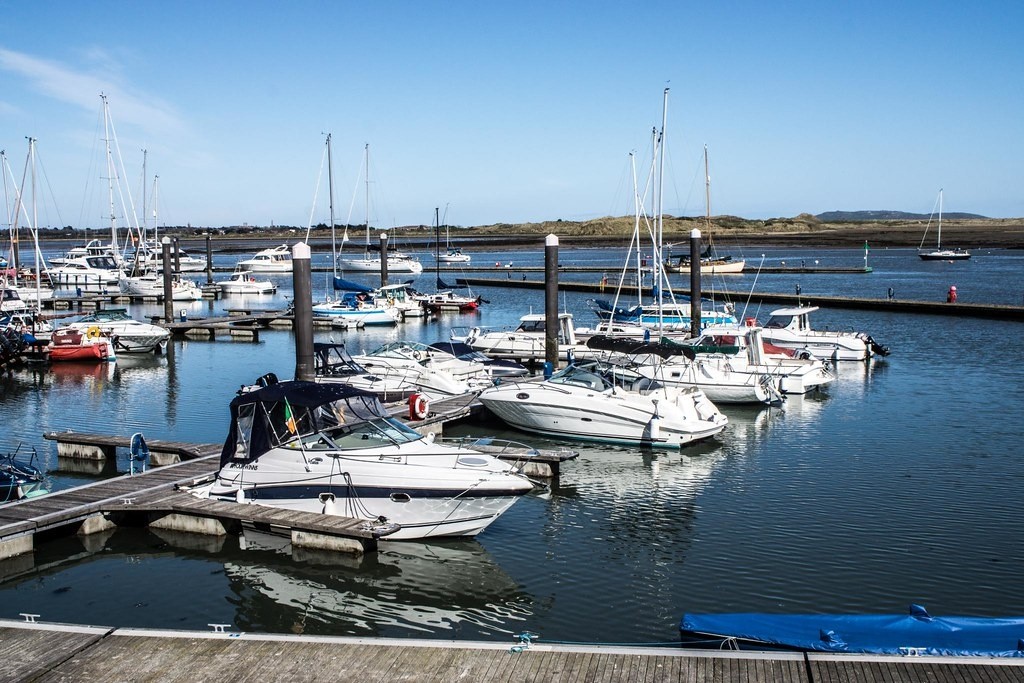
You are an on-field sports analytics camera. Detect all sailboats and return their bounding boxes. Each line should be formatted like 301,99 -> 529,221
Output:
302,132 -> 400,328
435,204 -> 470,263
591,84 -> 739,325
1,90 -> 214,313
337,141 -> 422,272
410,208 -> 481,311
916,187 -> 972,261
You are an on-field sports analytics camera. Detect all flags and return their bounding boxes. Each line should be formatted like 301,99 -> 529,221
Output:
284,404 -> 296,436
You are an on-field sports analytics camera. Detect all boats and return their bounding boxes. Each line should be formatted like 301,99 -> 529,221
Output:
371,282 -> 431,318
477,360 -> 729,449
597,364 -> 787,404
62,314 -> 169,353
622,350 -> 836,395
216,270 -> 276,294
239,245 -> 294,271
656,254 -> 746,273
237,362 -> 452,420
678,603 -> 1023,659
1,315 -> 55,340
711,305 -> 876,361
451,312 -> 625,360
429,341 -> 529,376
0,454 -> 49,504
44,332 -> 117,361
315,339 -> 494,392
187,372 -> 533,539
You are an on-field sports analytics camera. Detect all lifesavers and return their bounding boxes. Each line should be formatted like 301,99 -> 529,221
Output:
415,395 -> 429,419
86,326 -> 99,339
